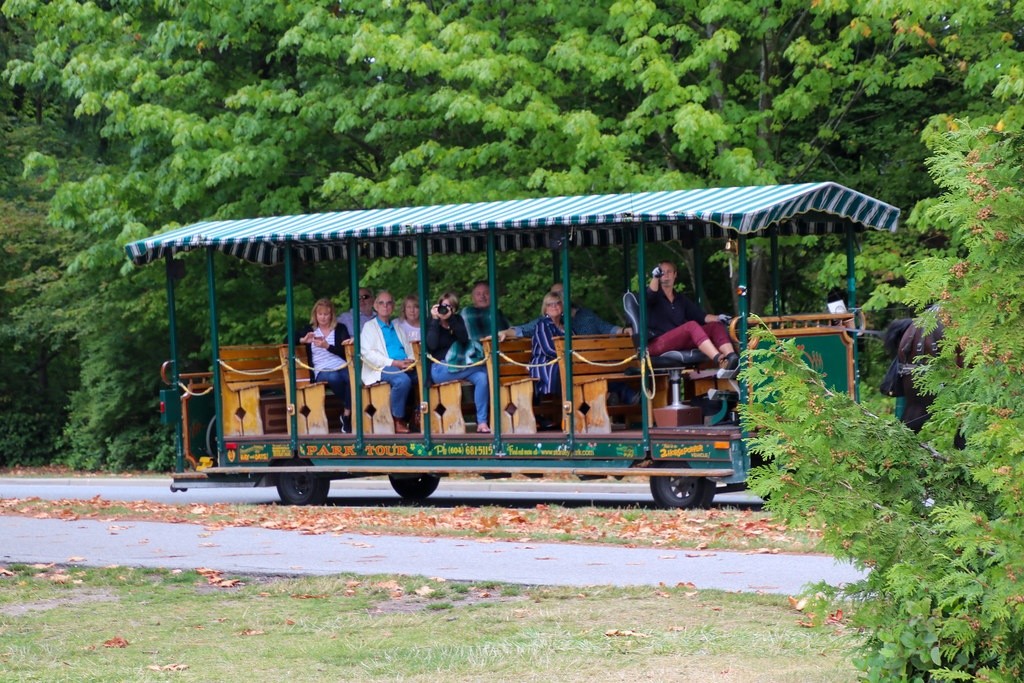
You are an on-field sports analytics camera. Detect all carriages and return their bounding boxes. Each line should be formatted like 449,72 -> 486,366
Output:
122,181 -> 974,510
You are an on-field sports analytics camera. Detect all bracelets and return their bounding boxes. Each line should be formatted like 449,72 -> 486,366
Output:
622,327 -> 625,334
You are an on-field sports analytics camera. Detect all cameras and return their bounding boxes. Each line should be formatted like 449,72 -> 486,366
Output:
438,303 -> 449,314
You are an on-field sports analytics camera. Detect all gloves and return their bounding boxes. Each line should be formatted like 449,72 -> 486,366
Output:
719,314 -> 732,322
652,263 -> 664,278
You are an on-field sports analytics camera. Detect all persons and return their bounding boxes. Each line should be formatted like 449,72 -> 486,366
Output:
645,261 -> 740,371
336,287 -> 377,345
481,280 -> 634,428
359,282 -> 507,433
283,299 -> 354,433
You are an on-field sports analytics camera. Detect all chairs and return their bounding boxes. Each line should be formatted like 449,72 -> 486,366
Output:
623,291 -> 712,409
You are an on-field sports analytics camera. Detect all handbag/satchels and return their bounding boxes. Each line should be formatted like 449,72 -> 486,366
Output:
445,341 -> 484,373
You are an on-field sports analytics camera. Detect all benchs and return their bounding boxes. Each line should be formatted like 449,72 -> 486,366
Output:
216,331 -> 669,437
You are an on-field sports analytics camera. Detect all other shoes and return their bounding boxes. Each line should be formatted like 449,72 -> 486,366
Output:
719,352 -> 738,370
477,423 -> 491,433
395,421 -> 410,433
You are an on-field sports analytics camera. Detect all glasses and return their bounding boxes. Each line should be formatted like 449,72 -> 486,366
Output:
376,300 -> 392,306
547,300 -> 562,309
359,294 -> 371,300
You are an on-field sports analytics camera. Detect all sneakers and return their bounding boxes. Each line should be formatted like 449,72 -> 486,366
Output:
340,414 -> 352,433
